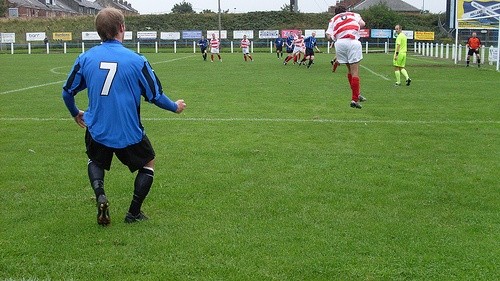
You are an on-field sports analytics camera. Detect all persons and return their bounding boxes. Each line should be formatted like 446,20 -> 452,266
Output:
393,24 -> 411,86
274,33 -> 284,59
466,32 -> 482,68
240,35 -> 254,61
197,35 -> 209,60
329,41 -> 341,72
209,33 -> 222,62
284,30 -> 320,69
62,7 -> 188,226
326,6 -> 366,108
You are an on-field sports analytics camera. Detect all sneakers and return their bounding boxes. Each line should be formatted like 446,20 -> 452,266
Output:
96,195 -> 110,225
406,78 -> 411,85
350,99 -> 362,108
124,210 -> 149,223
359,95 -> 366,101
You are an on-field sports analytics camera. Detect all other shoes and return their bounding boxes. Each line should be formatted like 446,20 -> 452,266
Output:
283,60 -> 315,69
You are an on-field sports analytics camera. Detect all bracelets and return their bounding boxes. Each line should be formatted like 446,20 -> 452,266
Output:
396,52 -> 399,55
